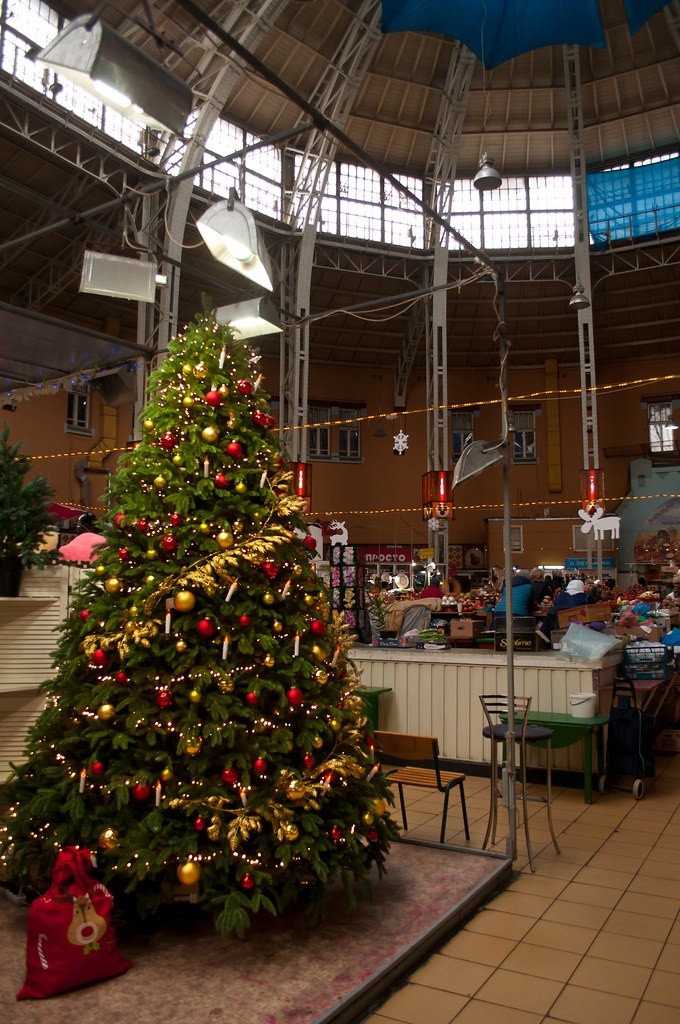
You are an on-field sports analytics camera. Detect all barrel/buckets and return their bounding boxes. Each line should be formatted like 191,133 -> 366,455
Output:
570,693 -> 596,718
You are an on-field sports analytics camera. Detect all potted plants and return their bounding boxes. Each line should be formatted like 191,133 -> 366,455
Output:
0,420 -> 64,597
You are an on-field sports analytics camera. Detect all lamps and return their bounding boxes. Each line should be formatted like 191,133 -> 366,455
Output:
474,151 -> 502,191
451,438 -> 505,492
34,0 -> 195,140
569,282 -> 591,310
371,420 -> 388,437
212,293 -> 285,340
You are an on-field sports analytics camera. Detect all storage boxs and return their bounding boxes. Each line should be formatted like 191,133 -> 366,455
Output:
550,629 -> 568,652
450,619 -> 484,639
652,729 -> 680,753
623,643 -> 675,680
647,694 -> 680,722
494,616 -> 542,652
601,626 -> 662,642
558,602 -> 613,628
378,630 -> 401,648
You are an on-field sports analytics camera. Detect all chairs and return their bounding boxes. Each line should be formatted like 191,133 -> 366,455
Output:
373,729 -> 469,845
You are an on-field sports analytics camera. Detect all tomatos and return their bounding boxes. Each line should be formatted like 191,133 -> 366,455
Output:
463,600 -> 483,611
608,594 -> 638,606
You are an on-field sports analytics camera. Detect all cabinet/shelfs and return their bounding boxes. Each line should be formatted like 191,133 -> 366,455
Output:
0,563 -> 68,785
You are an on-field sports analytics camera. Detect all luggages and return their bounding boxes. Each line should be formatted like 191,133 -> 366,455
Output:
599,678 -> 656,799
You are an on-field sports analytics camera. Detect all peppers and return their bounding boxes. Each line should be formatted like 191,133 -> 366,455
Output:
420,628 -> 450,643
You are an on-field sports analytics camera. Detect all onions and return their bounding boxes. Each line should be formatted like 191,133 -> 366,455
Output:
441,595 -> 458,604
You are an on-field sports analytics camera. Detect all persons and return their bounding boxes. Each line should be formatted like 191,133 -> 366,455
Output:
540,580 -> 594,649
489,569 -> 540,638
530,567 -> 553,604
550,569 -> 566,591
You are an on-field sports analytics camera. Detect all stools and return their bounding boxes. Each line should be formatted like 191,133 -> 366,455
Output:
478,693 -> 562,874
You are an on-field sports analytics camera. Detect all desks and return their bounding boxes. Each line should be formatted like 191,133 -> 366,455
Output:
353,686 -> 393,763
496,708 -> 608,805
597,678 -> 665,712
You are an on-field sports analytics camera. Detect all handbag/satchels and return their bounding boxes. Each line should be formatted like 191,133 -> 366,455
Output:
16,846 -> 134,1001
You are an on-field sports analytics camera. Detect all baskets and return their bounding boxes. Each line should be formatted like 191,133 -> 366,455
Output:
619,646 -> 669,679
